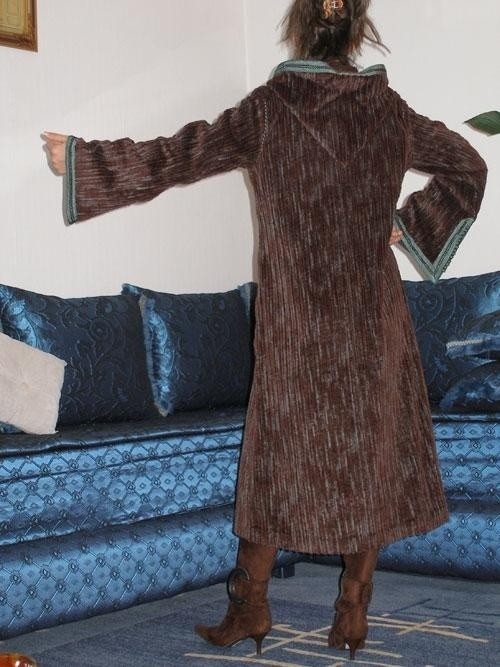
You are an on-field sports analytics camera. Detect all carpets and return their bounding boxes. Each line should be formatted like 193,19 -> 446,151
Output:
29,597 -> 497,667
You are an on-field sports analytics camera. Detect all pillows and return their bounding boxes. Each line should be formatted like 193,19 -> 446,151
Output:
438,364 -> 500,413
442,309 -> 500,362
400,270 -> 500,406
0,330 -> 68,435
122,283 -> 251,417
0,284 -> 143,434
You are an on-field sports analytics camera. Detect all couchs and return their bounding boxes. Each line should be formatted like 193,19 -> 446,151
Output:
0,271 -> 500,640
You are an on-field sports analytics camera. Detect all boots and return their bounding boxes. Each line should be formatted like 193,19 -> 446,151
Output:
194,539 -> 278,658
328,548 -> 378,659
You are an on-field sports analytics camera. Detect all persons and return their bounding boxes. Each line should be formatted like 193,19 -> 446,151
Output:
39,0 -> 488,660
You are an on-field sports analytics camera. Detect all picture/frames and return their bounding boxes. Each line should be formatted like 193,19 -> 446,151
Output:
0,0 -> 38,53
